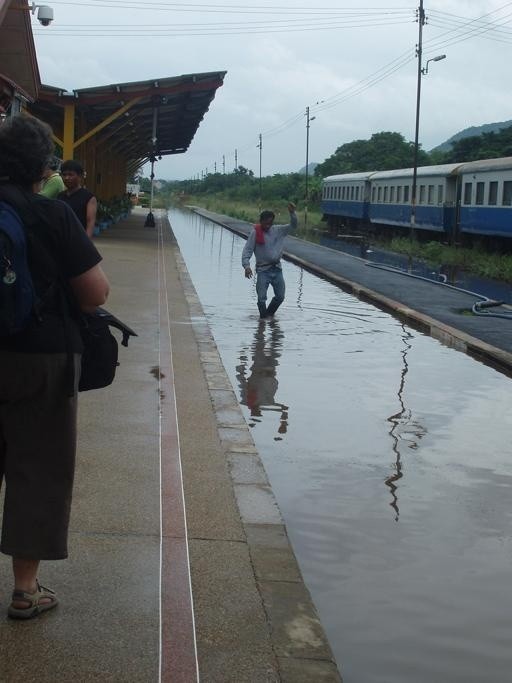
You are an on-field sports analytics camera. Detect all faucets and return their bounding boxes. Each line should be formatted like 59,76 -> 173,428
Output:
8,586 -> 59,619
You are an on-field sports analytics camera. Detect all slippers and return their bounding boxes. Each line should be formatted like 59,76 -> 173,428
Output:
0,195 -> 57,342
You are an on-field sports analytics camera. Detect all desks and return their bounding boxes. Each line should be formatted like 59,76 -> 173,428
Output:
305,116 -> 316,199
410,53 -> 447,243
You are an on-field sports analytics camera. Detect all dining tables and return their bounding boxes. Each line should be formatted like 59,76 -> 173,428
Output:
93,192 -> 133,236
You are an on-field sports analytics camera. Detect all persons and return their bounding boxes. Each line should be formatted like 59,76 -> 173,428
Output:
241,201 -> 298,317
0,111 -> 110,617
37,155 -> 64,200
56,159 -> 97,240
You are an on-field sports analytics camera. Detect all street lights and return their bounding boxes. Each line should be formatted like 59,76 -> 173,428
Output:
74,306 -> 138,392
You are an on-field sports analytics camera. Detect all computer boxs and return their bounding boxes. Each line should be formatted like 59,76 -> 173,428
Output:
36,5 -> 54,25
